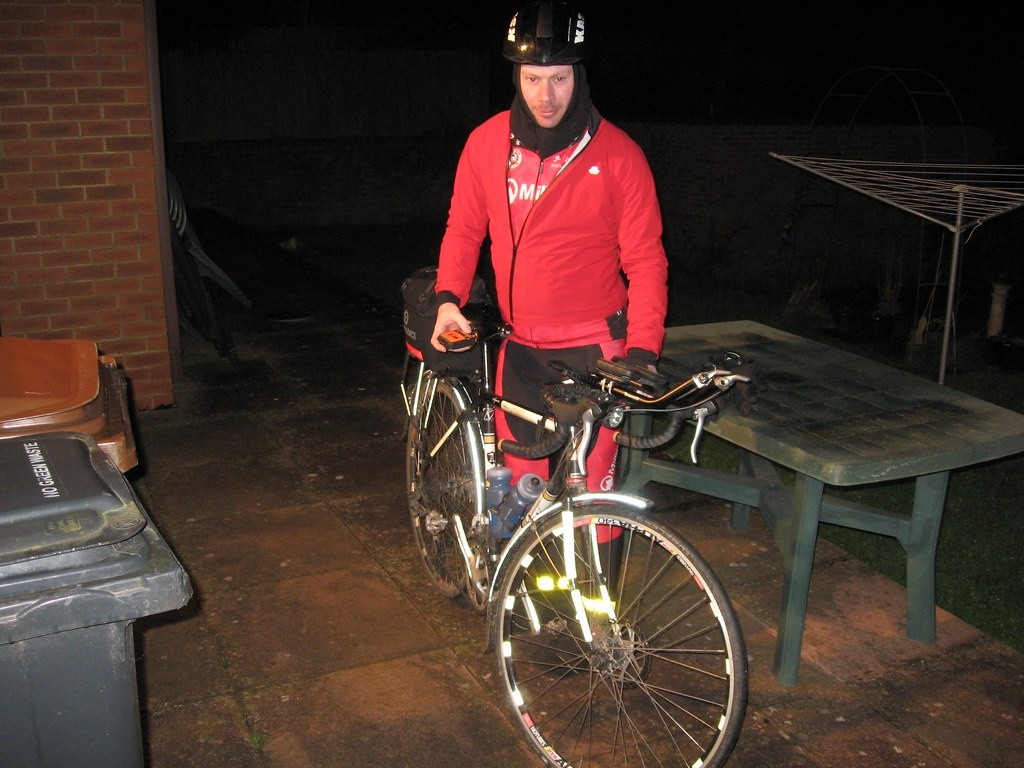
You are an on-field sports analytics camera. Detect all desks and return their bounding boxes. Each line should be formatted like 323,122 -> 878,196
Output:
614,320 -> 1024,689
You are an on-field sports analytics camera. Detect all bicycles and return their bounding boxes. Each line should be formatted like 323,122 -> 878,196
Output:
398,266 -> 751,768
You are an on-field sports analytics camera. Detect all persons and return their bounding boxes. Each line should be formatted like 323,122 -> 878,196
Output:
429,1 -> 669,678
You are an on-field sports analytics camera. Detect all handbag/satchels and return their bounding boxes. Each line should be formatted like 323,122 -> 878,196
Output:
399,264 -> 494,381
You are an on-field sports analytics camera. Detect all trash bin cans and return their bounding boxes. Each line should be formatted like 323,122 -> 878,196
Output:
0,428 -> 196,767
0,330 -> 143,474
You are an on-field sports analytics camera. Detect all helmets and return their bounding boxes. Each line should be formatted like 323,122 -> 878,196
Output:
501,0 -> 591,66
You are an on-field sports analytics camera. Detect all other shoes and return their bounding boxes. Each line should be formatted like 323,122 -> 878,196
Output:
535,610 -> 592,677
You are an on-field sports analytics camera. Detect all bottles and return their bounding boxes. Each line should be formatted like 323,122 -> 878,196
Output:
490,473 -> 544,539
487,468 -> 511,513
